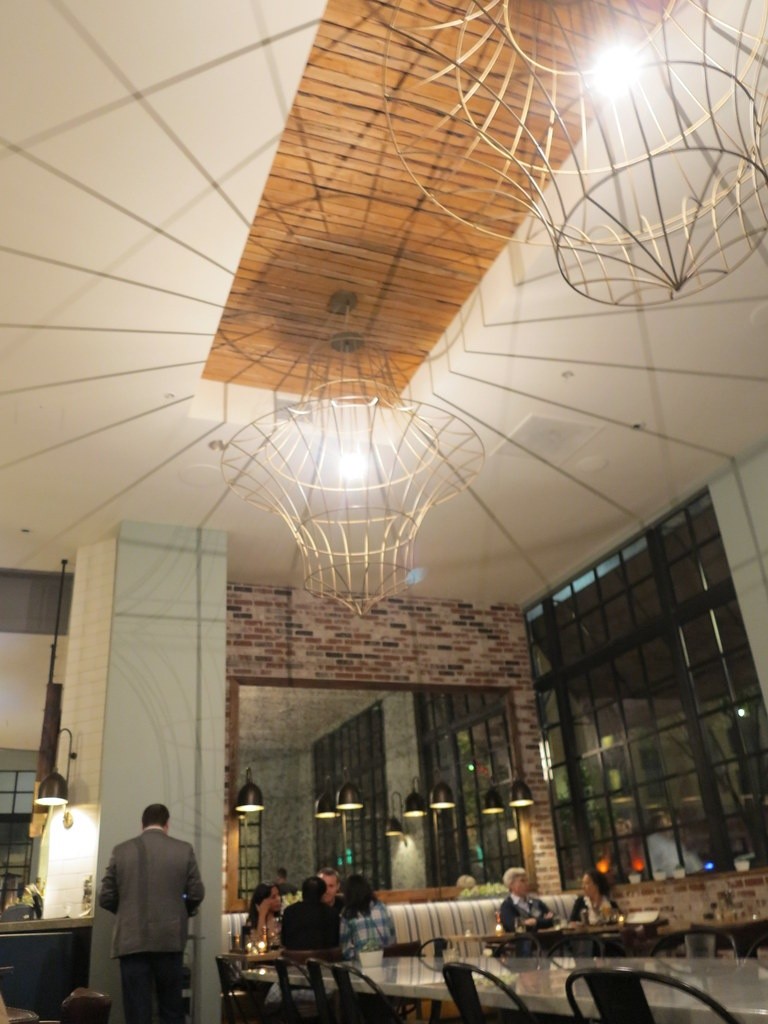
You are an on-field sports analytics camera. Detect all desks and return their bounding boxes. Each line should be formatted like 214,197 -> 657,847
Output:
238,954 -> 768,1024
476,918 -> 647,960
222,948 -> 281,975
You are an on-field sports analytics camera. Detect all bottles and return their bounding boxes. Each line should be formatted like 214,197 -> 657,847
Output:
494,911 -> 506,937
261,926 -> 270,953
685,931 -> 717,976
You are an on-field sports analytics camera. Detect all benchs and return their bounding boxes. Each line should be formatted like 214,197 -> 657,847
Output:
385,893 -> 579,958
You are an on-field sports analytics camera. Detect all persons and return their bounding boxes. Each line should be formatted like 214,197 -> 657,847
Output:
98,803 -> 205,1024
571,871 -> 623,959
499,867 -> 562,958
239,867 -> 396,1024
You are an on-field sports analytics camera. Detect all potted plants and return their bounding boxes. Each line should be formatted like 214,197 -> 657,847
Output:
653,867 -> 666,882
627,871 -> 642,884
733,856 -> 750,871
672,865 -> 685,880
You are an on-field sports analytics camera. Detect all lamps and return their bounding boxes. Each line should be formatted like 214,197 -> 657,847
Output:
234,767 -> 265,821
381,1 -> 768,303
315,767 -> 537,844
213,290 -> 485,617
34,727 -> 77,828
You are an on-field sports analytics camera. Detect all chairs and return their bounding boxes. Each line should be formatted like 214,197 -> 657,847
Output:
218,924 -> 768,1024
